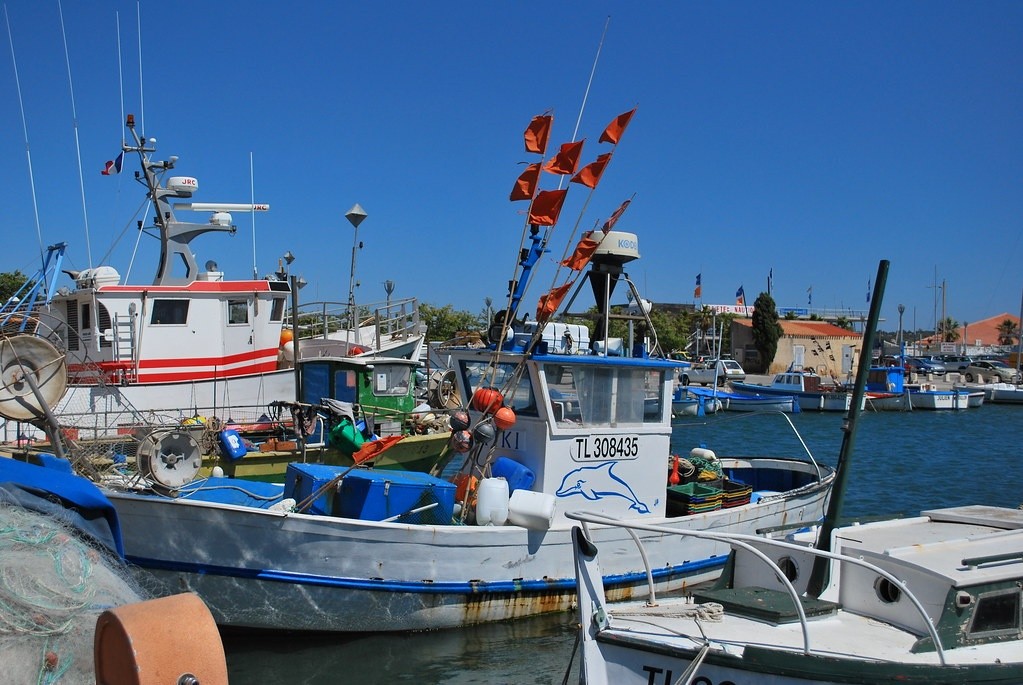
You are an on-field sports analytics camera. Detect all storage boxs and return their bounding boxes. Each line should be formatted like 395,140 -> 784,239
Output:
667,479 -> 753,515
283,463 -> 458,526
198,272 -> 224,281
260,442 -> 297,451
374,422 -> 401,438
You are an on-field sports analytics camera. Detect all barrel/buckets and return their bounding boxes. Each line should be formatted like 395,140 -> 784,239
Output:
476,456 -> 557,528
217,428 -> 247,461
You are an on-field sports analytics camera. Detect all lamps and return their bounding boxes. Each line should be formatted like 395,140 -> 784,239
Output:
629,299 -> 651,316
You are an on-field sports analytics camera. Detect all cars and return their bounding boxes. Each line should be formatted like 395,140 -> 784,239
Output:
965,360 -> 1023,383
978,356 -> 1009,363
670,351 -> 693,361
691,355 -> 713,363
871,355 -> 973,376
720,353 -> 733,360
679,359 -> 745,386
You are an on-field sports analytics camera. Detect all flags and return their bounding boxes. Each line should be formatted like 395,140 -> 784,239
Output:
736,286 -> 743,306
807,287 -> 811,305
866,278 -> 871,302
694,273 -> 701,297
509,108 -> 630,326
101,151 -> 123,175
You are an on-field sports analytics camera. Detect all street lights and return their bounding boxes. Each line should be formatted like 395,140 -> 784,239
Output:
925,284 -> 946,343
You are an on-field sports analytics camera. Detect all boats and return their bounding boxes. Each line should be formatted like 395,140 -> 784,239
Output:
831,366 -> 968,409
729,363 -> 867,412
414,317 -> 793,417
0,113 -> 476,490
566,499 -> 1022,684
954,381 -> 1023,408
93,232 -> 837,634
